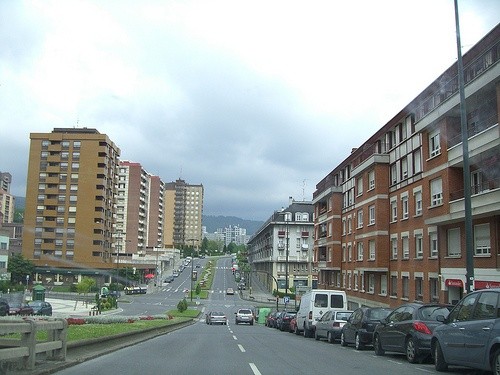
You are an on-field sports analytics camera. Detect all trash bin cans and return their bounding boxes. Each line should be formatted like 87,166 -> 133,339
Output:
101,287 -> 108,295
32,284 -> 46,302
255,308 -> 271,325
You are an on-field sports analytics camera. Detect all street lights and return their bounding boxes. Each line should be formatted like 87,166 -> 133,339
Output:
186,238 -> 199,301
278,212 -> 289,311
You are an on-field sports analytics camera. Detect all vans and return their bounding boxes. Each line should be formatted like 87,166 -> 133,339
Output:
295,289 -> 347,338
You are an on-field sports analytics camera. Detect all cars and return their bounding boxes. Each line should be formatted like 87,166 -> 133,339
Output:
31,301 -> 52,316
265,310 -> 297,334
234,309 -> 254,325
0,297 -> 9,316
173,272 -> 178,277
431,288 -> 500,375
8,302 -> 33,316
371,302 -> 456,364
339,307 -> 396,350
226,288 -> 231,295
315,310 -> 355,344
239,283 -> 245,290
205,311 -> 226,325
165,276 -> 174,282
